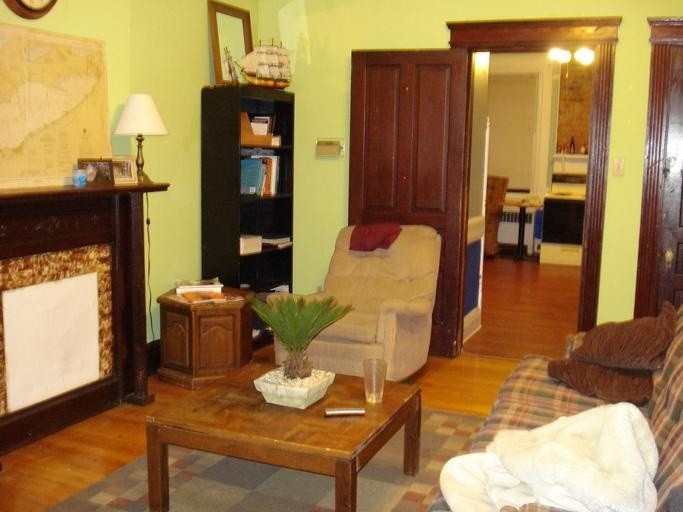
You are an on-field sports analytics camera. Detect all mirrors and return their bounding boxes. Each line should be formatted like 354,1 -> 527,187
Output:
206,0 -> 256,89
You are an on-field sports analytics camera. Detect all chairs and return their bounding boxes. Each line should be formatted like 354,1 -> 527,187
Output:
485,174 -> 510,257
264,220 -> 444,385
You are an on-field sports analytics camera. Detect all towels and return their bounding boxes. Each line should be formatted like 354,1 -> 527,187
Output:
347,220 -> 401,253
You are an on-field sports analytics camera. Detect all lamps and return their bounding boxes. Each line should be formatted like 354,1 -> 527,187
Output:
111,93 -> 169,185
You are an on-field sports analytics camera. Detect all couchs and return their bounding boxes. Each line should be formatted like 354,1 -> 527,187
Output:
427,302 -> 683,511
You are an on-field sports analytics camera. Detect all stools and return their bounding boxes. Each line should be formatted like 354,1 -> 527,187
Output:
155,279 -> 256,388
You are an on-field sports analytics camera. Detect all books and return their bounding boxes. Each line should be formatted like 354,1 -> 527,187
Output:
240,113 -> 294,293
175,277 -> 245,303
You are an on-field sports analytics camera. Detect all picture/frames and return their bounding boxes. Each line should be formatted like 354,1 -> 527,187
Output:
78,157 -> 114,187
112,155 -> 138,185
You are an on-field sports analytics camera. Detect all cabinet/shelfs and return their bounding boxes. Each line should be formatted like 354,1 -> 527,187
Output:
630,13 -> 682,319
198,85 -> 296,351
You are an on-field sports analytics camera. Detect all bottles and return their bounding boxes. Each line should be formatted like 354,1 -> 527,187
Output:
580,136 -> 589,154
556,136 -> 576,154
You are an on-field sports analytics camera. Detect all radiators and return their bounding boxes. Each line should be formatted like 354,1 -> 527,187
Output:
495,205 -> 537,256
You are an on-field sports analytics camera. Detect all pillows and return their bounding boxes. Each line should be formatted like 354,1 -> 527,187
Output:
571,298 -> 677,373
547,356 -> 654,407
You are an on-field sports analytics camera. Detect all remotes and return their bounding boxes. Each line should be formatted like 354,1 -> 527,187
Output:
325,407 -> 365,416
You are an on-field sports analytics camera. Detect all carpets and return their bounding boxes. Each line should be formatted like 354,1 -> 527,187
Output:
48,404 -> 488,510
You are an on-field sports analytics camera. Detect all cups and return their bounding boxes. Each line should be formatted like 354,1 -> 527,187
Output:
72,169 -> 88,187
362,358 -> 387,404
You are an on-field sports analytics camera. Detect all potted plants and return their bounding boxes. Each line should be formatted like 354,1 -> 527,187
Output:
246,291 -> 356,410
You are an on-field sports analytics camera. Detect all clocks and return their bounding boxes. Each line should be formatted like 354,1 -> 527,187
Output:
3,1 -> 58,20
